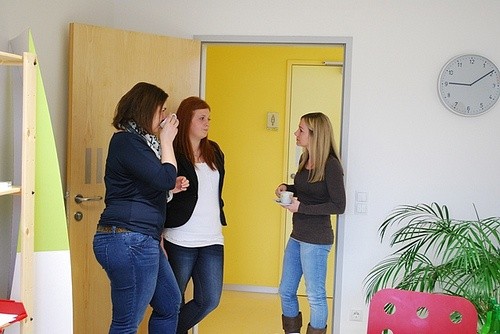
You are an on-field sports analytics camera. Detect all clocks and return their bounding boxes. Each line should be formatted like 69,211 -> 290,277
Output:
437,52 -> 500,117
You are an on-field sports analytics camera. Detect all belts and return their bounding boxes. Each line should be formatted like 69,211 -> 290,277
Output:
96,225 -> 131,233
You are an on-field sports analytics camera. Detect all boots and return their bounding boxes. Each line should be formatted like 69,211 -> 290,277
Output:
282,312 -> 302,334
305,323 -> 326,334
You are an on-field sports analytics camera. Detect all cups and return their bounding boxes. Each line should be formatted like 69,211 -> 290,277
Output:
160,114 -> 177,128
279,191 -> 294,203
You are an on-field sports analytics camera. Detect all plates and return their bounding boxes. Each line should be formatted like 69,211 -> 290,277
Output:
274,199 -> 292,205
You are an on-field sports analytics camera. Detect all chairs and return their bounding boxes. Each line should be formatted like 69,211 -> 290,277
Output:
367,288 -> 478,334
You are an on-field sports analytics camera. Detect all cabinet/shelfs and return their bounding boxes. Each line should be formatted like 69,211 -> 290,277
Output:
0,51 -> 37,334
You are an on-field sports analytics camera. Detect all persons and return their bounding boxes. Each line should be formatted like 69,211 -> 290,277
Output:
92,81 -> 182,334
275,112 -> 346,334
162,97 -> 227,334
